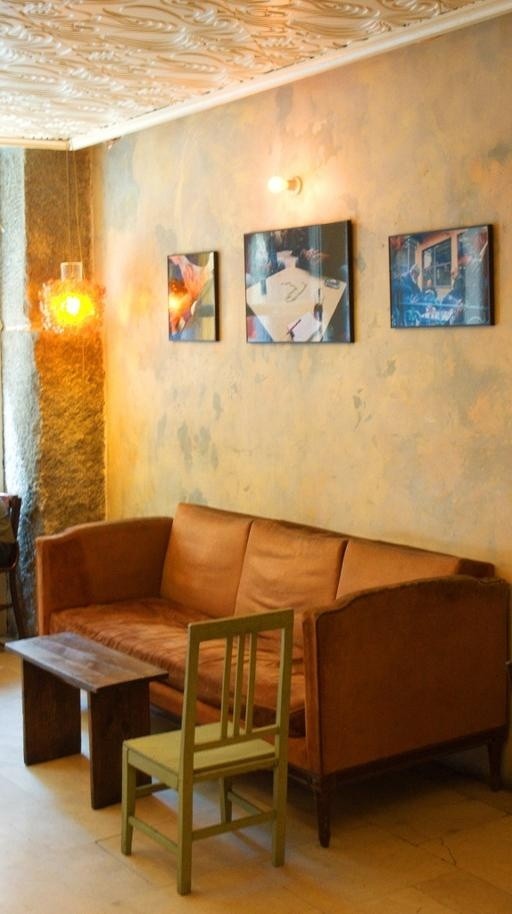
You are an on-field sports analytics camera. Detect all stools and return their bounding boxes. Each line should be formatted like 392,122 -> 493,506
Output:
6,632 -> 168,808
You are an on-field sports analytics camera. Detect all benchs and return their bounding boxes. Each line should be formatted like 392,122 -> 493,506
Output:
34,500 -> 512,848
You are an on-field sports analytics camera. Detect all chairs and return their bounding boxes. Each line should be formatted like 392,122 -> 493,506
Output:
120,605 -> 294,896
0,493 -> 27,647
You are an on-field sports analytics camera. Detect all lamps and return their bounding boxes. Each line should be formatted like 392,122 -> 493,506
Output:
38,262 -> 105,335
267,174 -> 302,198
